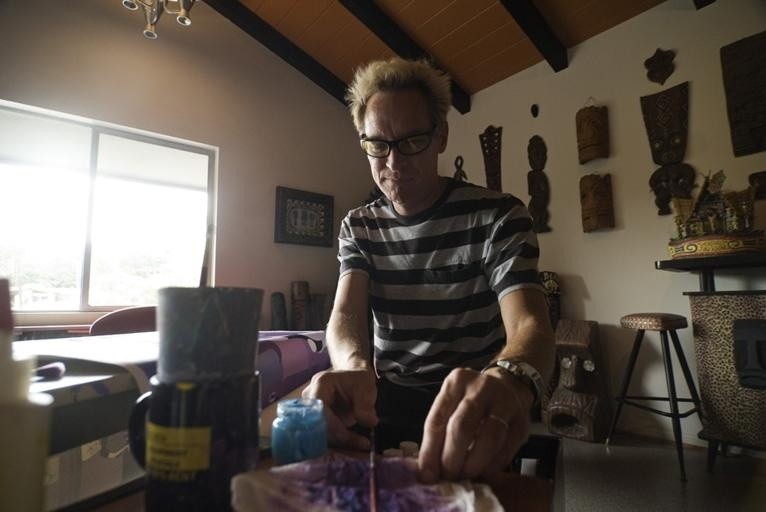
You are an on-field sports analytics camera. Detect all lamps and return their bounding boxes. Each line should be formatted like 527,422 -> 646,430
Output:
120,1 -> 196,39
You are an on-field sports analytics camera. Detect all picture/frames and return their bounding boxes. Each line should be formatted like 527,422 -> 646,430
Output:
273,184 -> 335,250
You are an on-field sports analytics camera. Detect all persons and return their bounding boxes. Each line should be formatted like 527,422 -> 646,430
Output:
300,59 -> 560,484
526,134 -> 552,233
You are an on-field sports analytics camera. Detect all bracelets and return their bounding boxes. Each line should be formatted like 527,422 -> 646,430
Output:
486,355 -> 543,407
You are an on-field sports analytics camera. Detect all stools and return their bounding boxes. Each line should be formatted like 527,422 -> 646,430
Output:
605,314 -> 706,484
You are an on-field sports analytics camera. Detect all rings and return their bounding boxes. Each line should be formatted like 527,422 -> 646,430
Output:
487,410 -> 513,428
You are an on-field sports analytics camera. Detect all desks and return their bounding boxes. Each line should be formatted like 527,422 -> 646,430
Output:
653,248 -> 766,479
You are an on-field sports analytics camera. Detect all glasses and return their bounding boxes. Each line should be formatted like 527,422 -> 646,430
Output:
360,125 -> 436,158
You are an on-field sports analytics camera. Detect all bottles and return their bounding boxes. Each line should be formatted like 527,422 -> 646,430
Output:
270,398 -> 328,464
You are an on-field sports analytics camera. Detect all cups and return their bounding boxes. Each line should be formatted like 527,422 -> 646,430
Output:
141,370 -> 261,512
